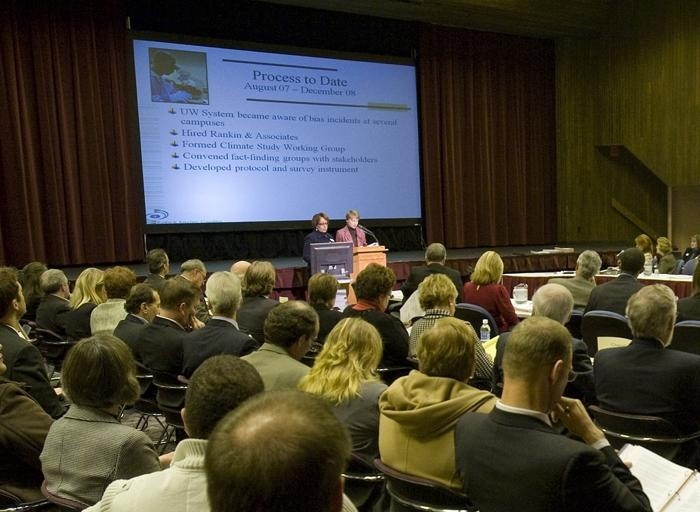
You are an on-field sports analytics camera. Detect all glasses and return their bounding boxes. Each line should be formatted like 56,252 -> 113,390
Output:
318,222 -> 328,226
563,362 -> 578,383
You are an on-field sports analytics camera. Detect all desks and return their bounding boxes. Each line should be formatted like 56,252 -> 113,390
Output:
384,290 -> 534,322
501,271 -> 693,299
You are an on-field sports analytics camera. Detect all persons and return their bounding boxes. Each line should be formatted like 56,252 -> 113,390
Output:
377,317 -> 497,507
294,272 -> 345,363
377,317 -> 497,510
455,317 -> 654,511
410,275 -> 494,384
38,335 -> 165,498
492,284 -> 597,403
226,261 -> 248,292
544,251 -> 600,311
0,265 -> 64,423
18,265 -> 46,308
78,355 -> 358,511
335,265 -> 410,366
37,270 -> 72,352
238,300 -> 321,395
0,343 -> 54,480
180,271 -> 255,379
405,245 -> 462,303
238,259 -> 279,340
147,49 -> 194,102
90,264 -> 129,342
451,250 -> 516,335
337,210 -> 366,248
302,212 -> 333,266
590,284 -> 700,441
57,267 -> 106,343
652,236 -> 675,272
141,250 -> 169,294
138,277 -> 197,390
288,316 -> 389,474
682,235 -> 698,269
205,387 -> 351,512
155,259 -> 210,322
107,286 -> 160,368
633,234 -> 655,272
583,250 -> 645,326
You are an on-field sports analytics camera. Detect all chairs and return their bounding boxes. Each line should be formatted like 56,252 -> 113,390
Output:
148,369 -> 188,455
454,302 -> 500,340
494,380 -> 509,392
406,356 -> 420,370
370,457 -> 470,512
673,318 -> 700,354
580,307 -> 634,357
374,367 -> 411,385
133,358 -> 166,431
18,317 -> 41,331
28,327 -> 71,389
340,451 -> 387,512
39,478 -> 92,512
0,489 -> 57,512
177,374 -> 189,386
564,308 -> 585,341
587,407 -> 700,461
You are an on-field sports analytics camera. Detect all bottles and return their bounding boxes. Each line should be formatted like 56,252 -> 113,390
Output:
644,258 -> 653,276
653,255 -> 658,268
481,320 -> 490,341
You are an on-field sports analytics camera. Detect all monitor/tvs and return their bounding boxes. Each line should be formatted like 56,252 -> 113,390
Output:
309,242 -> 353,280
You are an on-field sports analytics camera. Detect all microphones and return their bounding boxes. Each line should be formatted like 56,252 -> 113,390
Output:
358,224 -> 379,246
325,232 -> 334,242
